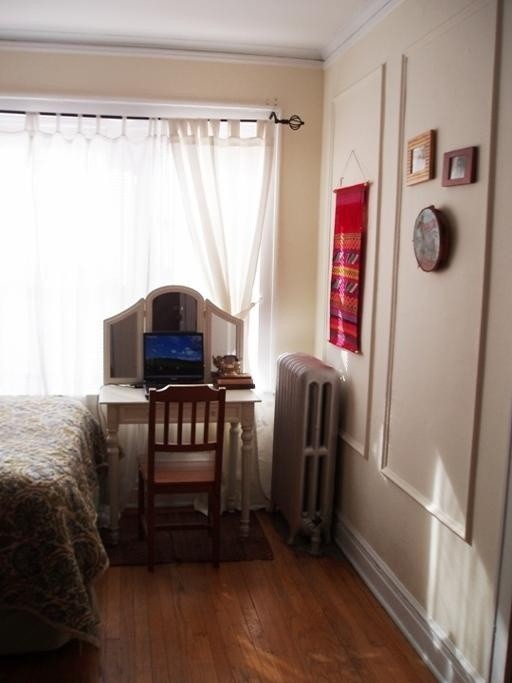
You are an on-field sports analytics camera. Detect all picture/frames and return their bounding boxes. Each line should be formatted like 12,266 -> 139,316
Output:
404,127 -> 477,190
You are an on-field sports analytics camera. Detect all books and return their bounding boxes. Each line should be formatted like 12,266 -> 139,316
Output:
210,371 -> 252,384
213,380 -> 255,389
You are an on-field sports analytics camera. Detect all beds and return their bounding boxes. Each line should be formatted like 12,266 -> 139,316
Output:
1,391 -> 115,652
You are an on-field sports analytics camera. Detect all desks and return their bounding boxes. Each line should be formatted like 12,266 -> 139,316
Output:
97,383 -> 264,547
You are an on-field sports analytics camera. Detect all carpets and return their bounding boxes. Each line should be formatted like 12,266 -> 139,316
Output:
93,503 -> 275,566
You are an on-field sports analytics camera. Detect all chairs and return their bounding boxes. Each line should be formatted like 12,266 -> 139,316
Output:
130,383 -> 229,570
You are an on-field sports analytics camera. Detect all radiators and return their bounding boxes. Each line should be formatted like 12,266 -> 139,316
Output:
267,348 -> 345,557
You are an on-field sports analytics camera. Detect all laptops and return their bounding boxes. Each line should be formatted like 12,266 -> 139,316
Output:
143,332 -> 204,400
